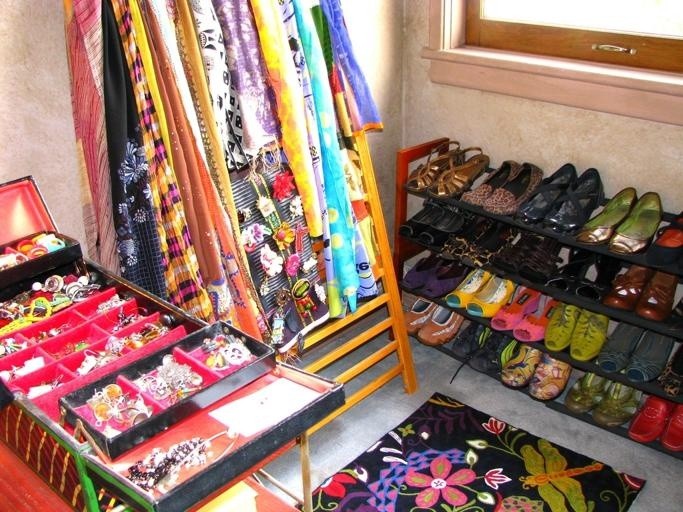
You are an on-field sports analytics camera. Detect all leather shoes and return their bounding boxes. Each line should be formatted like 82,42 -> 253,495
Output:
461,160 -> 683,264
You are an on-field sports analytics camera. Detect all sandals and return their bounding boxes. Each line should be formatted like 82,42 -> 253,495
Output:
425,147 -> 489,199
403,141 -> 464,194
399,199 -> 518,268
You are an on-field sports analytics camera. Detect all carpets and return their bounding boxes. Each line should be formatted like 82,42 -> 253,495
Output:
294,393 -> 647,512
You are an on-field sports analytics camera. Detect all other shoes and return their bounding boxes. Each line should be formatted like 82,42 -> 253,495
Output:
530,260 -> 682,453
402,256 -> 530,387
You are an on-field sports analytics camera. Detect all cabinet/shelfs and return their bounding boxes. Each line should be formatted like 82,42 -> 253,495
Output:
394,138 -> 683,462
64,0 -> 419,438
0,176 -> 347,512
